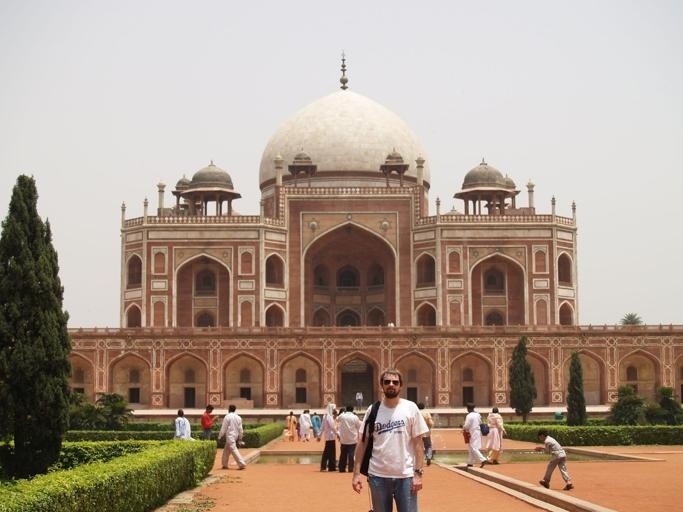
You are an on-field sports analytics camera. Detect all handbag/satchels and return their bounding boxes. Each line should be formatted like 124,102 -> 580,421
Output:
480,424 -> 490,436
360,433 -> 373,475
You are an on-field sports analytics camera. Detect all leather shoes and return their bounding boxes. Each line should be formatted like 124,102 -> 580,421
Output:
467,458 -> 497,469
319,462 -> 354,472
563,484 -> 573,491
540,480 -> 550,489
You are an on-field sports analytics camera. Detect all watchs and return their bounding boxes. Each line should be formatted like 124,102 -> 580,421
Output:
415,468 -> 424,475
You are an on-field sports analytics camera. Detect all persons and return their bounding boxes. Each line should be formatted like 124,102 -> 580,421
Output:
352,368 -> 429,512
486,407 -> 506,464
463,403 -> 487,468
417,402 -> 433,465
200,405 -> 219,440
533,429 -> 574,490
173,409 -> 196,441
218,405 -> 247,470
285,392 -> 364,472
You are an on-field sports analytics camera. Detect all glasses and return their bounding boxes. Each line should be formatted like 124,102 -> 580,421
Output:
383,380 -> 400,385
